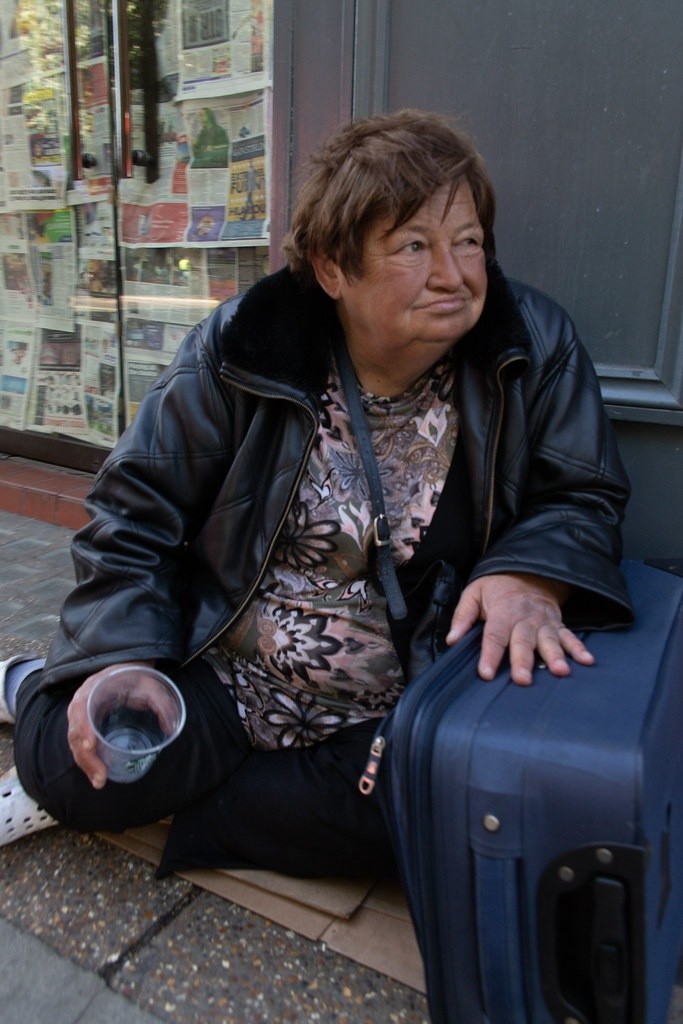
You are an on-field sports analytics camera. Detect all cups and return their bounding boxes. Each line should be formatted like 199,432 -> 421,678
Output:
85,666 -> 187,783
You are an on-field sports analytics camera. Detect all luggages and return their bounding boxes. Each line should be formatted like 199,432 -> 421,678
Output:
390,560 -> 683,1024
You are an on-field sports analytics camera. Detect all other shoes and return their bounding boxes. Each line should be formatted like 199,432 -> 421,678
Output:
0,655 -> 42,724
0,767 -> 60,846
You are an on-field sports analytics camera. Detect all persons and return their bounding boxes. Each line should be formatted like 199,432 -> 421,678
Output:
0,109 -> 631,880
